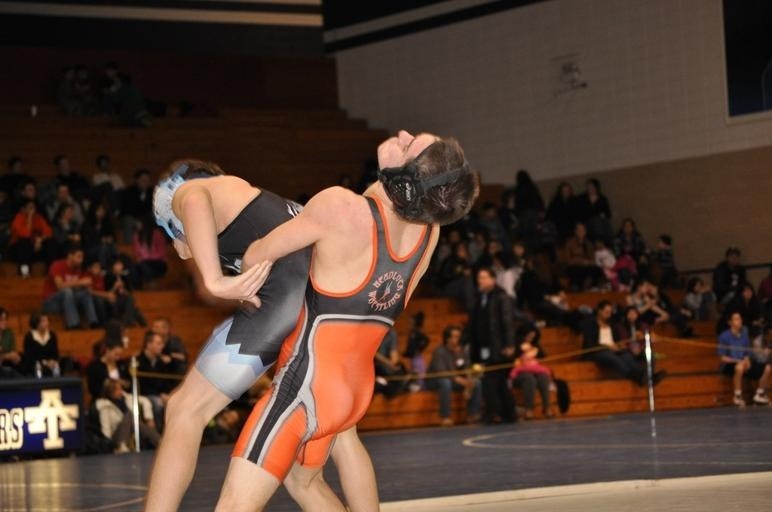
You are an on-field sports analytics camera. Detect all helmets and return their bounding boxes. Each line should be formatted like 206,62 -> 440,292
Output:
152,166 -> 218,240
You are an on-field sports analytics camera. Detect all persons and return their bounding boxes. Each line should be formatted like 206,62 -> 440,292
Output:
370,170 -> 772,425
144,158 -> 381,512
214,128 -> 481,512
1,155 -> 188,457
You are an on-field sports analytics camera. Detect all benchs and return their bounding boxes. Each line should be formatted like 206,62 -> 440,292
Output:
2,263 -> 725,397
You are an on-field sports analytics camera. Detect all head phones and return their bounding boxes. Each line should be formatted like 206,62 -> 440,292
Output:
386,162 -> 477,209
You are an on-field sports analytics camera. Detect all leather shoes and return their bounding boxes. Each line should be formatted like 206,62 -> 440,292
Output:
496,417 -> 518,425
653,369 -> 666,385
638,369 -> 646,386
475,417 -> 494,425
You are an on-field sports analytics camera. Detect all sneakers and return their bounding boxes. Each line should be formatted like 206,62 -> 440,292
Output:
733,393 -> 746,407
753,391 -> 768,405
